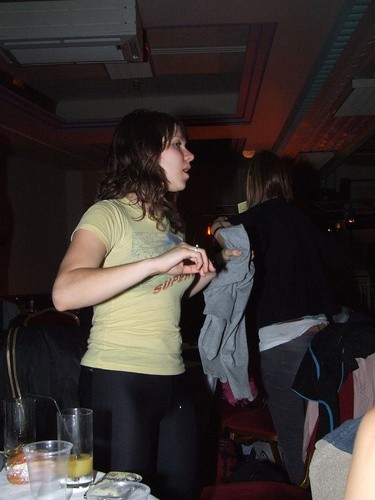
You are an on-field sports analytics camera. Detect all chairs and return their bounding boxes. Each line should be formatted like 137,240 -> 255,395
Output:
200,319 -> 355,500
6,310 -> 91,443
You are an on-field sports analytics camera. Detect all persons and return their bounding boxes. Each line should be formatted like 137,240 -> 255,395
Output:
227,150 -> 349,487
52,109 -> 255,500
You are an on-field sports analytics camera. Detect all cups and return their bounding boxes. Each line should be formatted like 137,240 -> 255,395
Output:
3,398 -> 37,485
23,440 -> 74,500
84,481 -> 150,500
56,408 -> 93,487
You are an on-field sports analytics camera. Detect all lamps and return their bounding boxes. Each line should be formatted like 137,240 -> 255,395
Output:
0,0 -> 145,67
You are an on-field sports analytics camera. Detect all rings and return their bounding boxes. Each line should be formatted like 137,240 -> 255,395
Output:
195,245 -> 198,252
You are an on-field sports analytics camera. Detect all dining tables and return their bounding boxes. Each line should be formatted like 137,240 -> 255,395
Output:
1,467 -> 160,500
